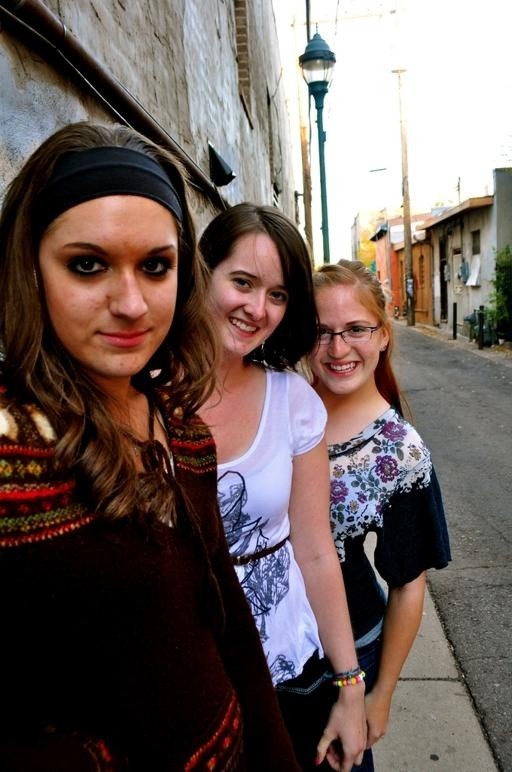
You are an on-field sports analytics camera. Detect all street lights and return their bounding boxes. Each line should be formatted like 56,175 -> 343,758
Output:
299,22 -> 335,263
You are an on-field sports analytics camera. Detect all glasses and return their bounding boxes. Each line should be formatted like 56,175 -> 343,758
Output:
317,326 -> 377,345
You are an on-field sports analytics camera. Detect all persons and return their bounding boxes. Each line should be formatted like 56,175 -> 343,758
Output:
300,258 -> 451,771
152,202 -> 368,771
0,117 -> 304,772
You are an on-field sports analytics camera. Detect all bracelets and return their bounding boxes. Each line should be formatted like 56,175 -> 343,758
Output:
331,668 -> 366,687
